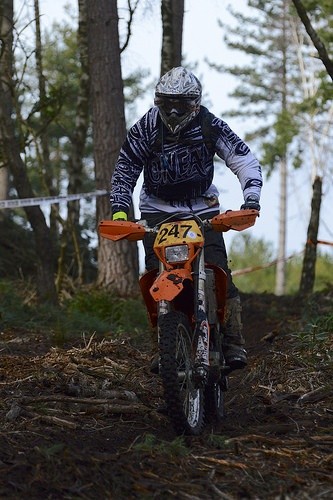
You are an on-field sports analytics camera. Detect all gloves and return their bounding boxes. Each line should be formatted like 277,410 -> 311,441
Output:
240,196 -> 260,211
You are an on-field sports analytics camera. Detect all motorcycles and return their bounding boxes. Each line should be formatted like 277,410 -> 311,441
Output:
98,209 -> 260,436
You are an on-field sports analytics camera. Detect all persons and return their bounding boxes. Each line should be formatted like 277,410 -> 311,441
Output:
109,66 -> 262,375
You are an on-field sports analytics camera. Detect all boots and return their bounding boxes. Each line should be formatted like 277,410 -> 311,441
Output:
221,295 -> 247,368
146,310 -> 158,373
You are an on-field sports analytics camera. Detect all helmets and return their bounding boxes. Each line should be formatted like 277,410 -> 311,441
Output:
153,67 -> 201,134
